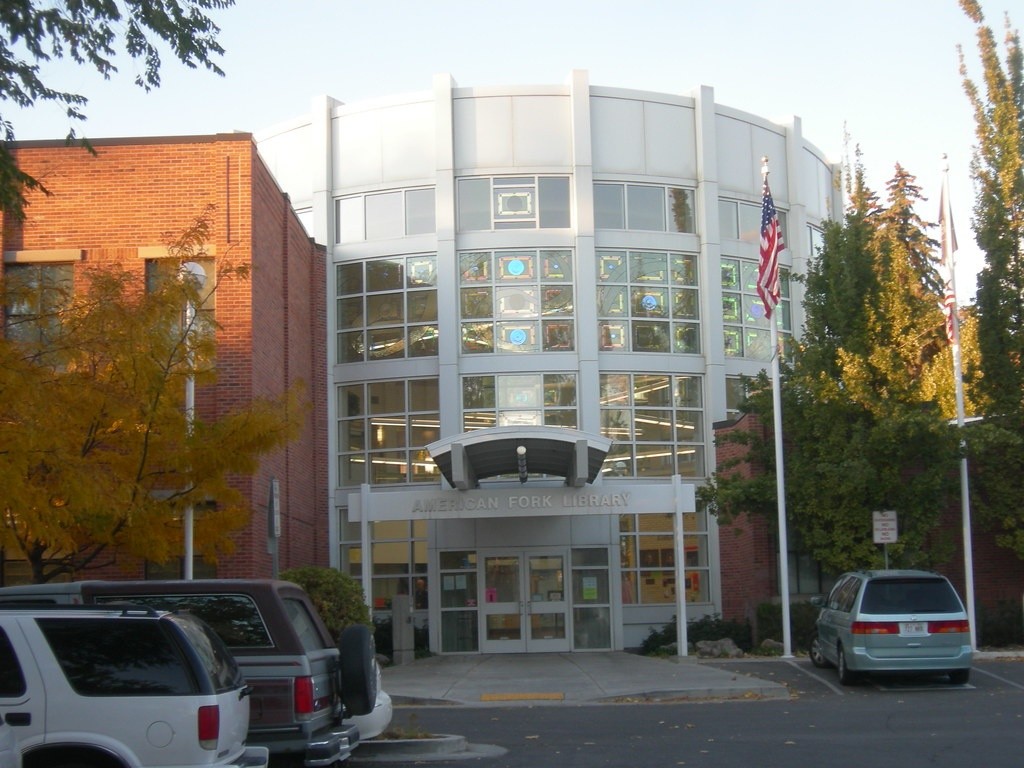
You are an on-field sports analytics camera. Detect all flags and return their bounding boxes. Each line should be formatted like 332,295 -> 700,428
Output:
757,176 -> 785,319
939,182 -> 958,343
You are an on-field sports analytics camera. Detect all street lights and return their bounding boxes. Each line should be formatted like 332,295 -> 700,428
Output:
175,261 -> 207,582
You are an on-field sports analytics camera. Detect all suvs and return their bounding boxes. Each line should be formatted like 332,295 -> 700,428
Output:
0,579 -> 379,767
806,569 -> 973,685
0,605 -> 273,767
